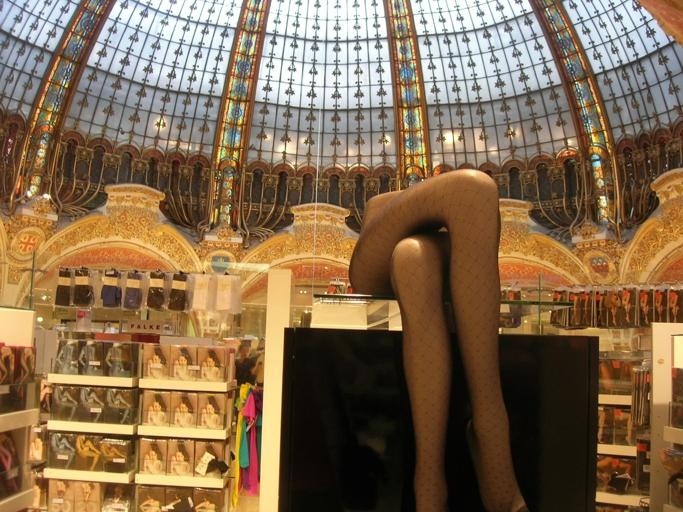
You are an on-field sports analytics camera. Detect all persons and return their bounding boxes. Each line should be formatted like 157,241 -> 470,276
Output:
0,345 -> 225,512
348,168 -> 531,512
250,336 -> 264,385
234,341 -> 255,420
553,288 -> 680,492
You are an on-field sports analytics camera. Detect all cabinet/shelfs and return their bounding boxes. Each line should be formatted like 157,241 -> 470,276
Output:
1,299 -> 683,512
